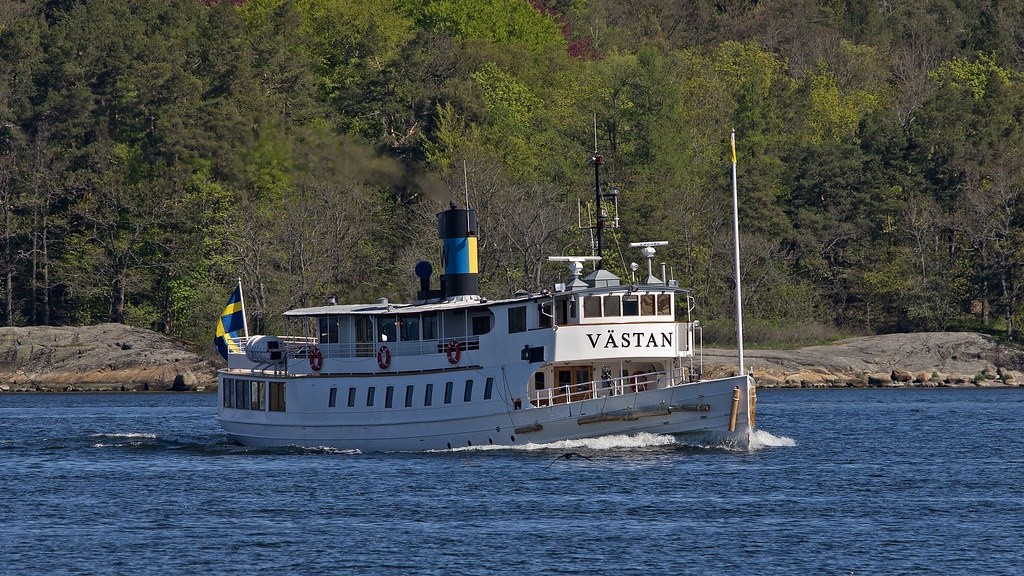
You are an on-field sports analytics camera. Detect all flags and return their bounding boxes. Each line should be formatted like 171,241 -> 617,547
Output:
214,285 -> 244,362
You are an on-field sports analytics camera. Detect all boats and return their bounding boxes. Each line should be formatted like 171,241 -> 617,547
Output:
216,111 -> 758,454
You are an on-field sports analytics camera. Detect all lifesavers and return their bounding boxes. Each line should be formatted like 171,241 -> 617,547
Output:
630,371 -> 647,391
447,340 -> 460,364
377,345 -> 391,369
310,347 -> 322,372
